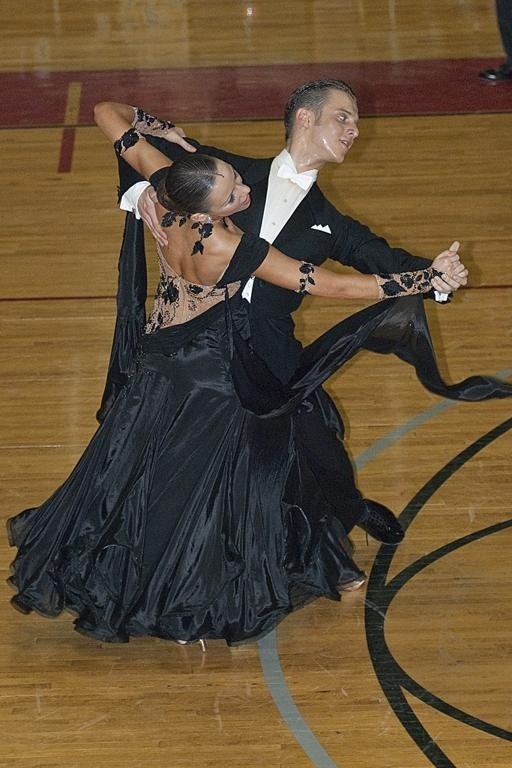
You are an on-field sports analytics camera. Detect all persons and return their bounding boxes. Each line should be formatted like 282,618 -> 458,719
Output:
115,76 -> 468,546
4,100 -> 470,645
476,0 -> 512,81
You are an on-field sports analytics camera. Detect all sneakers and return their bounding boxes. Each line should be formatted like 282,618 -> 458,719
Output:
354,499 -> 406,546
